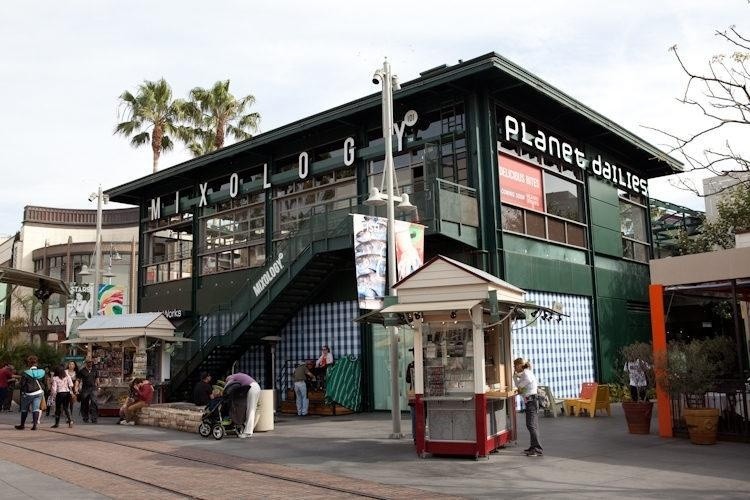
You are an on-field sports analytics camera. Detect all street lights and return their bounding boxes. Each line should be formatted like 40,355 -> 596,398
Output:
80,183 -> 121,363
364,55 -> 419,441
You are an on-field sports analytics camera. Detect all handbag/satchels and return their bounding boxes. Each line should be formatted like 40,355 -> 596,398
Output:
40,397 -> 47,410
70,391 -> 77,402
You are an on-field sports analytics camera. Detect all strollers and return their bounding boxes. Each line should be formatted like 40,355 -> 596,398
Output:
198,382 -> 252,440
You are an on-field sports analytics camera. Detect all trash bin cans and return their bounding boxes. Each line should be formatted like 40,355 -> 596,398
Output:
253,389 -> 274,432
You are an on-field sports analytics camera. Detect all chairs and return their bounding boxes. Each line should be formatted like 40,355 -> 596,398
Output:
305,357 -> 334,395
537,385 -> 569,418
575,385 -> 611,419
567,382 -> 601,416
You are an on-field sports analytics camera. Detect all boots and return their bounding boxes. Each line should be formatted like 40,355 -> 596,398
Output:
32,412 -> 38,430
66,415 -> 71,423
15,410 -> 28,429
52,416 -> 60,428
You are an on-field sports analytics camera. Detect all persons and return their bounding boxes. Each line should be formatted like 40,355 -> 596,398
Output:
68,292 -> 91,340
292,362 -> 316,416
512,358 -> 543,457
194,371 -> 214,405
0,355 -> 100,430
115,378 -> 154,426
223,372 -> 261,438
405,347 -> 426,448
623,358 -> 652,402
312,345 -> 334,391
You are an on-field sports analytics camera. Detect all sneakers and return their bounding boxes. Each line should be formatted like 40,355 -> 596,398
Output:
524,446 -> 543,457
120,420 -> 135,426
238,432 -> 252,438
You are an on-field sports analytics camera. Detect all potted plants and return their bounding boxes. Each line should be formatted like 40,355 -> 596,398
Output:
612,340 -> 663,435
659,336 -> 735,444
